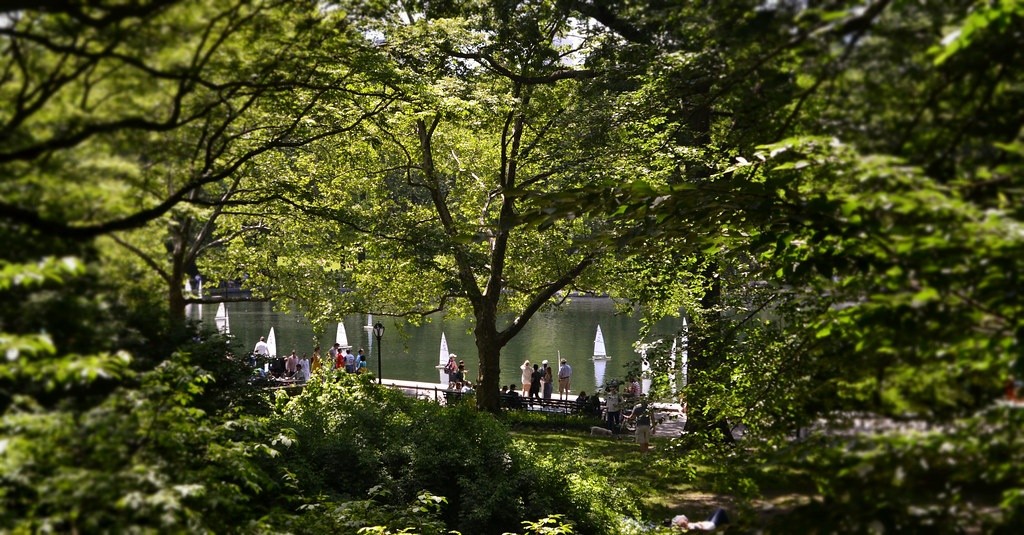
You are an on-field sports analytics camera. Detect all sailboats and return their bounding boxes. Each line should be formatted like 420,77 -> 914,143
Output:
592,324 -> 612,359
435,332 -> 451,369
215,302 -> 228,320
336,321 -> 352,349
364,313 -> 374,328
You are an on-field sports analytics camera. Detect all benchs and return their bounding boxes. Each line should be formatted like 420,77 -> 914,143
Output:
442,390 -> 529,411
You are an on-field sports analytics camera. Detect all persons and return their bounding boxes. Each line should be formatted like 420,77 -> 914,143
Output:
557,358 -> 572,401
356,356 -> 367,375
326,342 -> 339,366
311,347 -> 323,372
288,365 -> 305,386
444,352 -> 479,392
299,352 -> 311,381
253,336 -> 271,357
286,350 -> 299,374
670,505 -> 732,533
355,348 -> 365,365
343,350 -> 356,372
335,349 -> 344,367
502,357 -> 553,412
575,374 -> 657,455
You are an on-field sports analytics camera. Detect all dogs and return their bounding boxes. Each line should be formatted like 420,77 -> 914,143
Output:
590,426 -> 612,437
